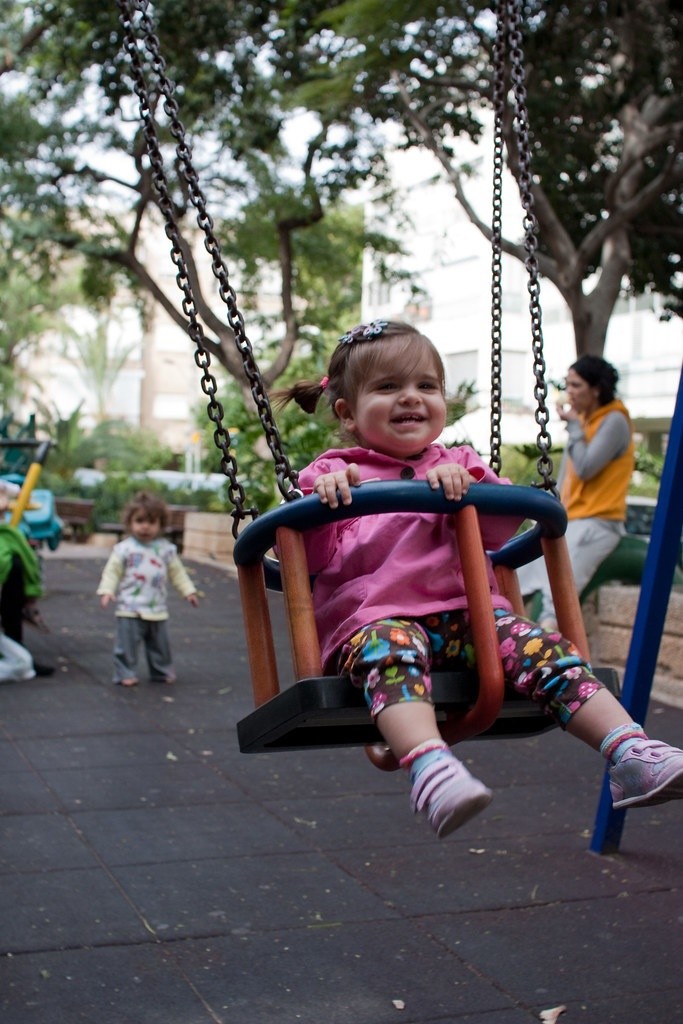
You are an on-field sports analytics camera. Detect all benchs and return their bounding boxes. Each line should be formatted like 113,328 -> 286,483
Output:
100,502 -> 197,550
53,496 -> 95,543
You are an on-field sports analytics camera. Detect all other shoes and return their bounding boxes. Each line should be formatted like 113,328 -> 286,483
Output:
121,677 -> 139,686
608,738 -> 683,809
408,755 -> 493,838
33,661 -> 55,676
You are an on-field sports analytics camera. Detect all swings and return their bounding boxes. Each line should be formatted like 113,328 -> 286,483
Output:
117,3 -> 629,759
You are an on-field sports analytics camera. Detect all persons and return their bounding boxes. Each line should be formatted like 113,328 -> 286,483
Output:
268,318 -> 683,837
95,492 -> 201,689
0,480 -> 60,678
513,352 -> 636,632
